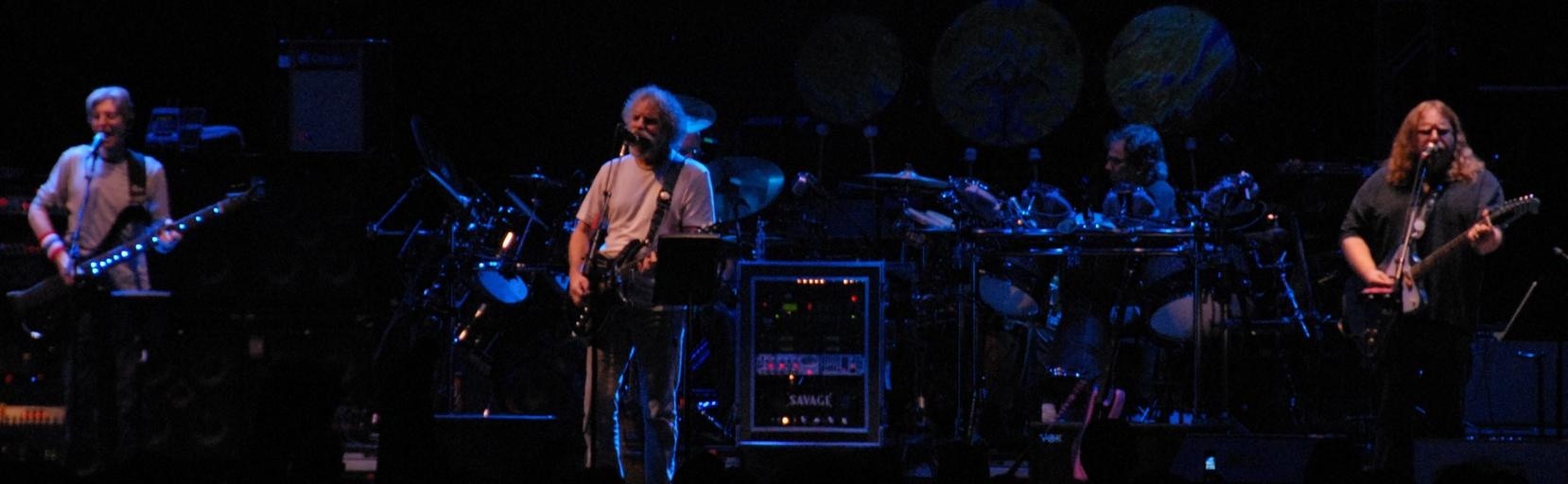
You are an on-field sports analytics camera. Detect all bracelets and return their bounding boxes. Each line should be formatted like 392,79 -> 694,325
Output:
37,229 -> 65,262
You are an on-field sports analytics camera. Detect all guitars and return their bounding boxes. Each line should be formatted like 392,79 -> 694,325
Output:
1335,195 -> 1543,358
575,226 -> 704,358
1,178 -> 272,427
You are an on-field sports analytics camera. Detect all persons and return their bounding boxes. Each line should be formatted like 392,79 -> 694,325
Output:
26,83 -> 186,468
567,84 -> 718,483
1046,122 -> 1183,423
1336,98 -> 1508,439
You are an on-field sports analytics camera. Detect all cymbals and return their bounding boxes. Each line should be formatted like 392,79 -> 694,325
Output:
426,167 -> 466,206
505,190 -> 546,228
667,94 -> 717,136
701,154 -> 785,224
475,261 -> 530,306
863,165 -> 948,190
969,222 -> 1055,237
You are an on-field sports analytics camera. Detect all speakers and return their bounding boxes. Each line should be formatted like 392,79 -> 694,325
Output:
1461,336 -> 1559,444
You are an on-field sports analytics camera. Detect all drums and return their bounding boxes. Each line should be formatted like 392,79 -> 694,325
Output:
1141,268 -> 1226,350
977,251 -> 1048,327
1031,283 -> 1141,379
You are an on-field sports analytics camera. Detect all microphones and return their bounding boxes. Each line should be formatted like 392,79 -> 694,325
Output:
615,123 -> 639,146
1426,142 -> 1442,155
89,131 -> 107,157
